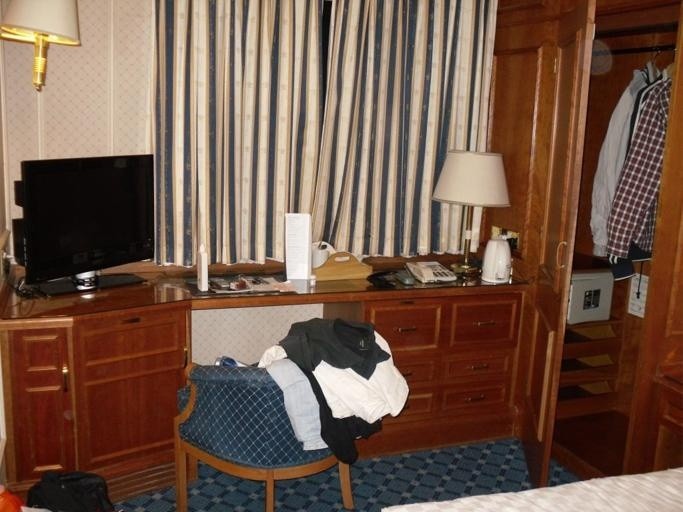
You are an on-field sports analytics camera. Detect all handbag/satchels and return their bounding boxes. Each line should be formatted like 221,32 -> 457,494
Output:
26,469 -> 114,511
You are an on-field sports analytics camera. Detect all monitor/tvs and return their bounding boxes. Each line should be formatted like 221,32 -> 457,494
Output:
20,153 -> 155,300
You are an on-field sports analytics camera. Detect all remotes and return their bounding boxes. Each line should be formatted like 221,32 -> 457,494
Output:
395,271 -> 415,286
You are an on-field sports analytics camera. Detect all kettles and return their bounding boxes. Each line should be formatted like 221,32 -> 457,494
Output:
481,233 -> 511,283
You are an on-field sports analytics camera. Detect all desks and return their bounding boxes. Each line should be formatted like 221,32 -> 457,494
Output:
144,263 -> 524,313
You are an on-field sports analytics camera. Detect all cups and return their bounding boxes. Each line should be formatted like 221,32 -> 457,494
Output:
311,249 -> 330,270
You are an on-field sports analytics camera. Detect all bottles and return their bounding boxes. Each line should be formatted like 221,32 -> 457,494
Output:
196,243 -> 209,292
215,356 -> 255,369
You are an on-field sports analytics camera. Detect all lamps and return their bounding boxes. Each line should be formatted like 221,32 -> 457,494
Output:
431,150 -> 511,277
0,0 -> 83,92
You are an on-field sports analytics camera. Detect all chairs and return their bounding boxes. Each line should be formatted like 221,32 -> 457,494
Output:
175,364 -> 359,512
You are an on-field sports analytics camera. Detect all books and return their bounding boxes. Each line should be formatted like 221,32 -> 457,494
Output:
240,276 -> 281,293
208,277 -> 252,294
245,276 -> 268,287
271,280 -> 302,293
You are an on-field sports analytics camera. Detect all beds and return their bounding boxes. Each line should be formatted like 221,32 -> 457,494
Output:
379,467 -> 682,512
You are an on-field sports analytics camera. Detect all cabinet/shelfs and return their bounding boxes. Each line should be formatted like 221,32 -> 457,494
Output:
73,311 -> 189,499
477,0 -> 683,487
7,324 -> 77,494
447,293 -> 518,442
324,297 -> 447,458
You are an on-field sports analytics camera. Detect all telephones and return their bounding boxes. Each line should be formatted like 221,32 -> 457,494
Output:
406,261 -> 457,284
